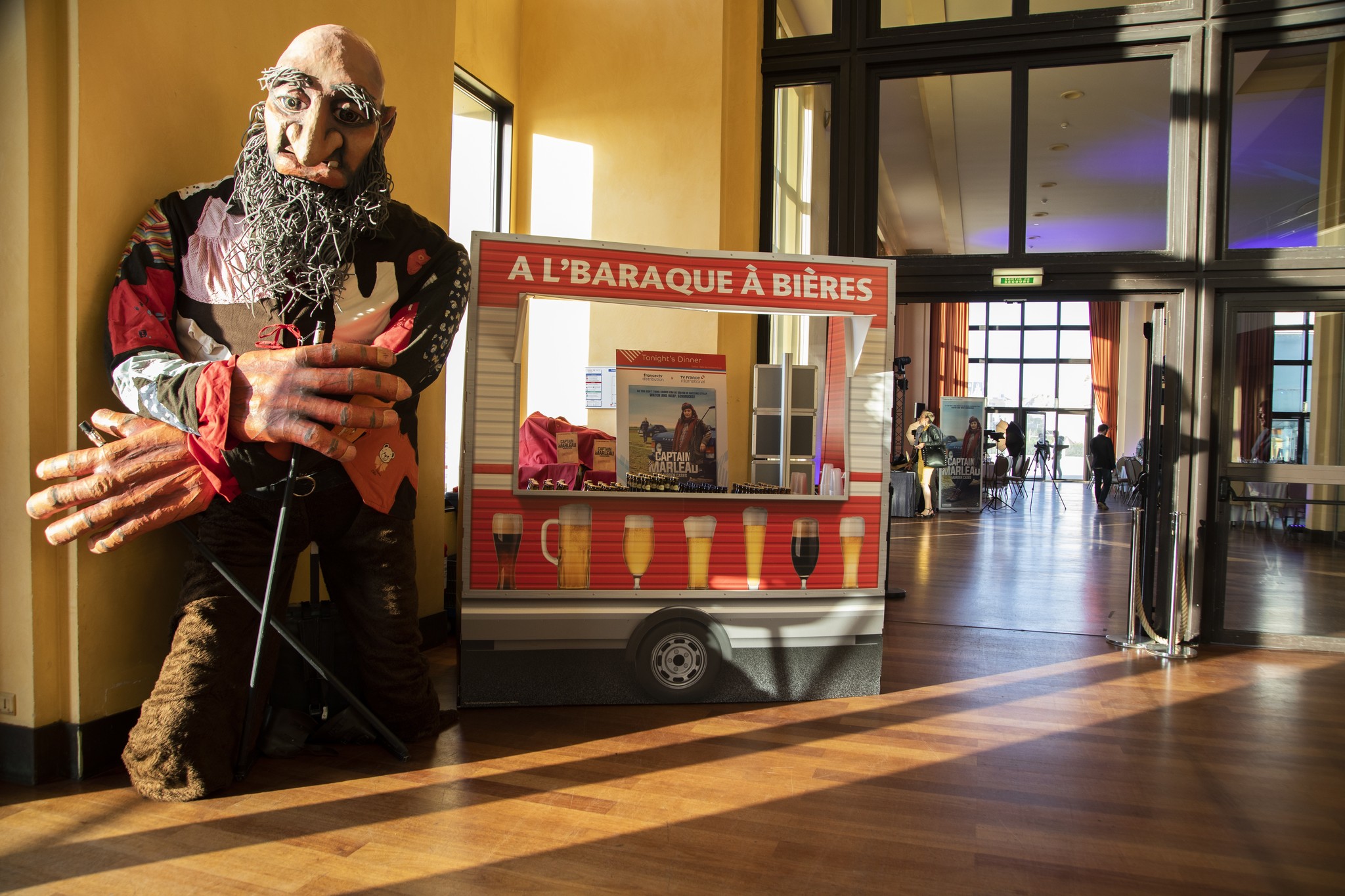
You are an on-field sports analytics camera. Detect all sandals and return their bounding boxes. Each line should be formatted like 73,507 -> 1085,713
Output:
918,508 -> 934,517
916,508 -> 931,519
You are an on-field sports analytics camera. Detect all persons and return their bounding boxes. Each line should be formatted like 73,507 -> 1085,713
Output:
1034,433 -> 1050,480
640,417 -> 648,444
904,411 -> 942,518
907,410 -> 941,516
1090,424 -> 1116,510
26,24 -> 474,797
1051,431 -> 1064,480
674,402 -> 712,484
1136,437 -> 1143,460
945,416 -> 982,503
1245,400 -> 1273,496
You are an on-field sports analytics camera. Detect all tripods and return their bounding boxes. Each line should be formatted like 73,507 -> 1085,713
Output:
1012,448 -> 1066,511
979,440 -> 1017,513
982,436 -> 995,503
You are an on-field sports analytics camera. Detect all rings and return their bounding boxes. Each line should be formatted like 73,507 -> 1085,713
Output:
918,447 -> 920,449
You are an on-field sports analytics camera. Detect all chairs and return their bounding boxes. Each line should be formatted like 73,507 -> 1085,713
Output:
1086,454 -> 1143,508
1229,481 -> 1256,530
982,452 -> 1030,503
1265,483 -> 1306,534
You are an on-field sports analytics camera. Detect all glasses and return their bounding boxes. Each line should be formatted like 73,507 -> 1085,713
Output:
920,416 -> 927,418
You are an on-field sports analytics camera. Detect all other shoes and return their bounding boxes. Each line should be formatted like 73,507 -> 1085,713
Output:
1097,505 -> 1102,508
1098,501 -> 1109,509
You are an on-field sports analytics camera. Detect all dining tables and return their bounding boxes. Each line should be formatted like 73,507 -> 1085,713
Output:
1230,482 -> 1304,522
1119,466 -> 1132,492
983,463 -> 1008,496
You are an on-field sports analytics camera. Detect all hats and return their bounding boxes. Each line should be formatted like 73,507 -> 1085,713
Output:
968,416 -> 981,431
680,402 -> 697,423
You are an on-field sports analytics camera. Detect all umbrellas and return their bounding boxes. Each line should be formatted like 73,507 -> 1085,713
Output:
995,419 -> 1026,458
905,420 -> 922,446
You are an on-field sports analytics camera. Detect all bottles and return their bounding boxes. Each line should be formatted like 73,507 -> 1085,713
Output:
983,450 -> 986,464
527,472 -> 791,494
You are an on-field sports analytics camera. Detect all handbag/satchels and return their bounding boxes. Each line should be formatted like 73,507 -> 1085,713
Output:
922,425 -> 947,468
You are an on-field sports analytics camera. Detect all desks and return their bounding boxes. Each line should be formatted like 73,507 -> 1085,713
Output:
890,471 -> 916,517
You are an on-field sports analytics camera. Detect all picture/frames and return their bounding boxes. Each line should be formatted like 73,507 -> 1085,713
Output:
936,396 -> 985,512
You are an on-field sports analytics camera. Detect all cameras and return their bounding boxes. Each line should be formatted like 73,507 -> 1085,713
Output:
1034,441 -> 1047,448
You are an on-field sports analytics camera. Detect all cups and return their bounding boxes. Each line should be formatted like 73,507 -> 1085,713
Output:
790,464 -> 844,496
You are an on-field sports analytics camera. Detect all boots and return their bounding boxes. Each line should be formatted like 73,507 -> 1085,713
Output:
949,488 -> 963,502
946,488 -> 957,502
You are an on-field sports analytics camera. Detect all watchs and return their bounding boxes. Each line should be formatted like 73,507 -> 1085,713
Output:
910,461 -> 914,465
